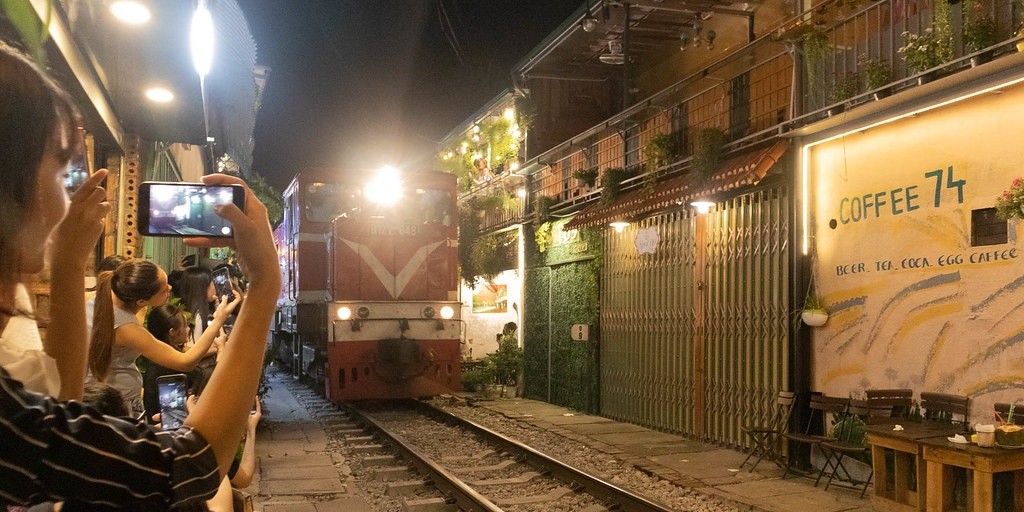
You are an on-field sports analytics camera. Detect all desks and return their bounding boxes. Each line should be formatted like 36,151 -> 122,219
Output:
864,418 -> 1024,512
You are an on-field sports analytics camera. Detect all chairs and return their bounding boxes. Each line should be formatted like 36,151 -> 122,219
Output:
920,392 -> 969,422
739,392 -> 798,472
820,399 -> 894,497
866,389 -> 912,418
994,403 -> 1024,425
782,393 -> 850,487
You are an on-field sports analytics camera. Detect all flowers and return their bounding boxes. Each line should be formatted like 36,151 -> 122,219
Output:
896,28 -> 934,76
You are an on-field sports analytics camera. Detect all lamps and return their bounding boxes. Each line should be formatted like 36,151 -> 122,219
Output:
690,192 -> 716,214
609,216 -> 631,233
680,13 -> 716,50
577,0 -> 598,32
599,40 -> 634,65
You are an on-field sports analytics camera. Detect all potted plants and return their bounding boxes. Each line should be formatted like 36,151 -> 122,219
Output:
572,169 -> 592,188
823,0 -> 1024,118
790,291 -> 828,326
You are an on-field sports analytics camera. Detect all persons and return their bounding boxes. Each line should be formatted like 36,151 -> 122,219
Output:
468,159 -> 509,189
501,322 -> 517,386
0,42 -> 282,512
174,199 -> 185,229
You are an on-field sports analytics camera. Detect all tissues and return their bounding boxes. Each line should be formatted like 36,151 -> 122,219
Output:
974,424 -> 996,448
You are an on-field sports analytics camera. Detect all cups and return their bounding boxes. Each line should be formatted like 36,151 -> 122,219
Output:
976,431 -> 995,447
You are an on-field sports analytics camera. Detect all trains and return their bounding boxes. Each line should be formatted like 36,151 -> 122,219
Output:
273,166 -> 466,404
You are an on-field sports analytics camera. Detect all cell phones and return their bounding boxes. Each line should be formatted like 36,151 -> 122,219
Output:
64,127 -> 91,198
155,374 -> 191,432
136,181 -> 246,238
210,267 -> 235,305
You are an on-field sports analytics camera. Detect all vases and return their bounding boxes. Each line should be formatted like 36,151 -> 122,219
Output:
918,75 -> 930,85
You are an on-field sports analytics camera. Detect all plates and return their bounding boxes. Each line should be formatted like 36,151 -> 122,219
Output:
995,441 -> 1024,449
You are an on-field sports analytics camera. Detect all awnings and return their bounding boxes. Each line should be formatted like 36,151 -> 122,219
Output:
563,140 -> 788,231
